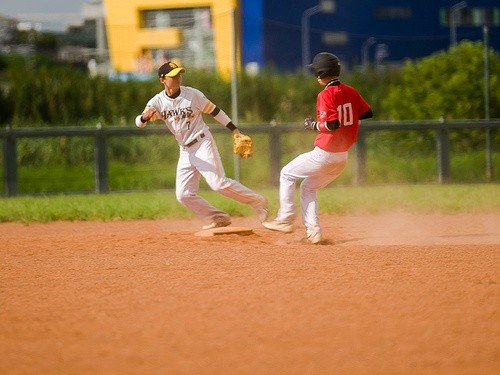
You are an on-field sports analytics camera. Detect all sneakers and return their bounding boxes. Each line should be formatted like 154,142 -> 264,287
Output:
202,220 -> 232,229
307,232 -> 323,243
257,200 -> 268,222
263,221 -> 293,232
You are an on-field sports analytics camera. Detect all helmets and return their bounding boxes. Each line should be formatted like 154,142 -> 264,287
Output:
306,52 -> 341,78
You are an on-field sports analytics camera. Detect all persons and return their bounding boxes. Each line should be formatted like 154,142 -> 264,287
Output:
262,52 -> 374,245
135,62 -> 269,231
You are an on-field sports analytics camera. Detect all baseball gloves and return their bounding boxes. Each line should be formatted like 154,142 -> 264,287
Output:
233,133 -> 253,158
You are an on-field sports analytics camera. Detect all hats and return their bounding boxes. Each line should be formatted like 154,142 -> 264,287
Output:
158,61 -> 185,78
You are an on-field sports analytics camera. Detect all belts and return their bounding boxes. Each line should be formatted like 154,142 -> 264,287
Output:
182,133 -> 205,149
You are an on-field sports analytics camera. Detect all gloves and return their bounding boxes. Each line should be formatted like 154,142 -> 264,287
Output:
304,117 -> 318,131
